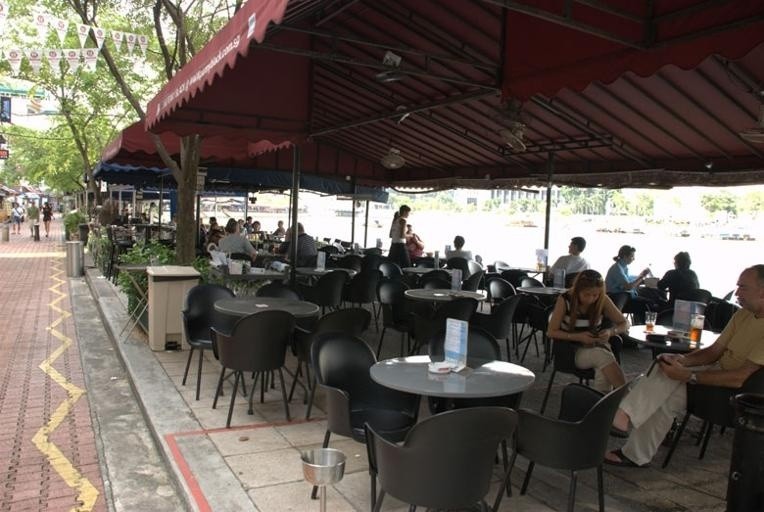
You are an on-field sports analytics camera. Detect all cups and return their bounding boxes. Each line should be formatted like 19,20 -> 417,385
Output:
688,313 -> 705,345
247,233 -> 261,242
645,312 -> 657,332
537,255 -> 546,271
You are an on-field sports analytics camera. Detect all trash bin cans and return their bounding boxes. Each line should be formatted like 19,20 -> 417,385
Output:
146,265 -> 202,351
65,242 -> 84,278
79,224 -> 89,246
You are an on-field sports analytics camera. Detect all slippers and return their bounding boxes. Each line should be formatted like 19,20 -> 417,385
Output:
603,448 -> 651,467
609,424 -> 629,438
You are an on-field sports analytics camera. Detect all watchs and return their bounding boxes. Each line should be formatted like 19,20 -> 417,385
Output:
689,371 -> 698,385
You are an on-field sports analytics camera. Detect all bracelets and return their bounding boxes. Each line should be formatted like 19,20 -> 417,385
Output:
565,332 -> 573,342
612,327 -> 619,338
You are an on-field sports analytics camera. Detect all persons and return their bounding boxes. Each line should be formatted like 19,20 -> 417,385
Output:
653,252 -> 700,325
110,201 -> 157,227
447,234 -> 473,262
387,206 -> 413,269
405,224 -> 425,259
605,245 -> 649,325
546,270 -> 631,401
546,237 -> 589,288
199,200 -> 318,271
605,264 -> 764,467
9,199 -> 54,240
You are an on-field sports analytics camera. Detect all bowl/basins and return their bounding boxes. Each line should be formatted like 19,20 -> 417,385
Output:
426,252 -> 433,257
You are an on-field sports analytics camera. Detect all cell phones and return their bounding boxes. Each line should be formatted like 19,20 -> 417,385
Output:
590,327 -> 600,341
